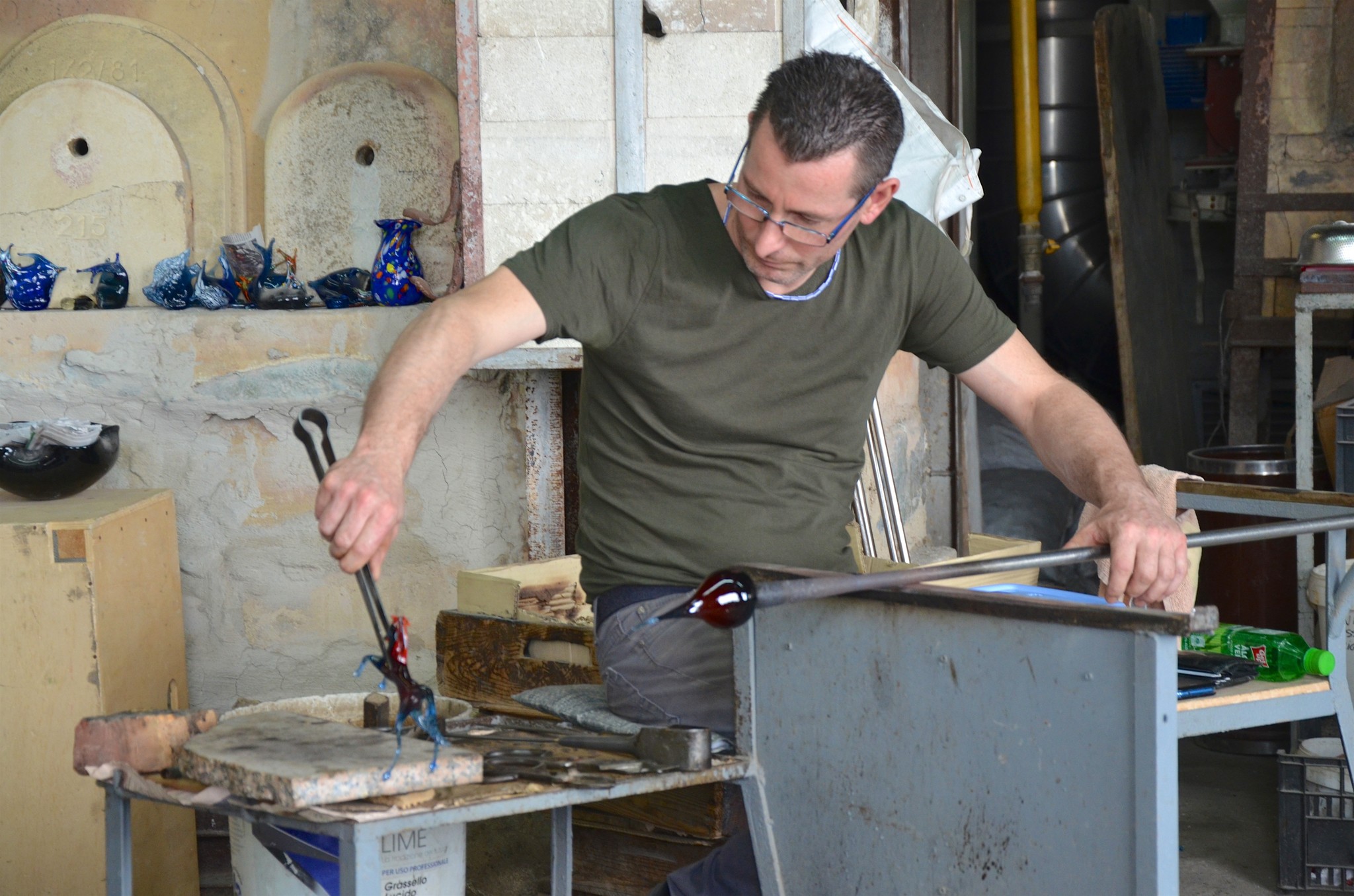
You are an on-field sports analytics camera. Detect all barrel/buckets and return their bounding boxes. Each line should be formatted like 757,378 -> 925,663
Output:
1186,445 -> 1332,633
1308,559 -> 1354,650
1296,738 -> 1354,885
220,692 -> 473,896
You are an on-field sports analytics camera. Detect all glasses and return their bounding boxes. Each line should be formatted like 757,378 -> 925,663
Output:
724,139 -> 878,247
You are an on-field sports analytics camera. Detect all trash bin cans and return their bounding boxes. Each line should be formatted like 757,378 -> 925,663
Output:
1187,443 -> 1297,633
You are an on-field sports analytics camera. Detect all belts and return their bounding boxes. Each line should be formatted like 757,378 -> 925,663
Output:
595,585 -> 699,631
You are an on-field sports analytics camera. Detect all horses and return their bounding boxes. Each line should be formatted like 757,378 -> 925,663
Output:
352,614 -> 456,779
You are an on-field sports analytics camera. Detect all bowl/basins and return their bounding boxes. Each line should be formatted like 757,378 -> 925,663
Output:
1289,224 -> 1354,265
0,421 -> 119,501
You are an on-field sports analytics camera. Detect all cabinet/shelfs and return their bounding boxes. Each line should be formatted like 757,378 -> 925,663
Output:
1184,46 -> 1243,171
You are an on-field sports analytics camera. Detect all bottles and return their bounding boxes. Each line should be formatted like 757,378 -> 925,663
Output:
372,219 -> 425,306
1181,623 -> 1335,681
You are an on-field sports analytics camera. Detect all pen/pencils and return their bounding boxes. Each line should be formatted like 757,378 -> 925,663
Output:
1177,686 -> 1217,700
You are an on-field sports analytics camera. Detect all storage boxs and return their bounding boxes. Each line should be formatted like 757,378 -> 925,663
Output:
229,815 -> 466,896
437,609 -> 724,841
1335,399 -> 1354,493
1274,715 -> 1354,895
458,525 -> 1042,663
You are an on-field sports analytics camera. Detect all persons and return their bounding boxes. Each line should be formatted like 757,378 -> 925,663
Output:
315,50 -> 1189,895
